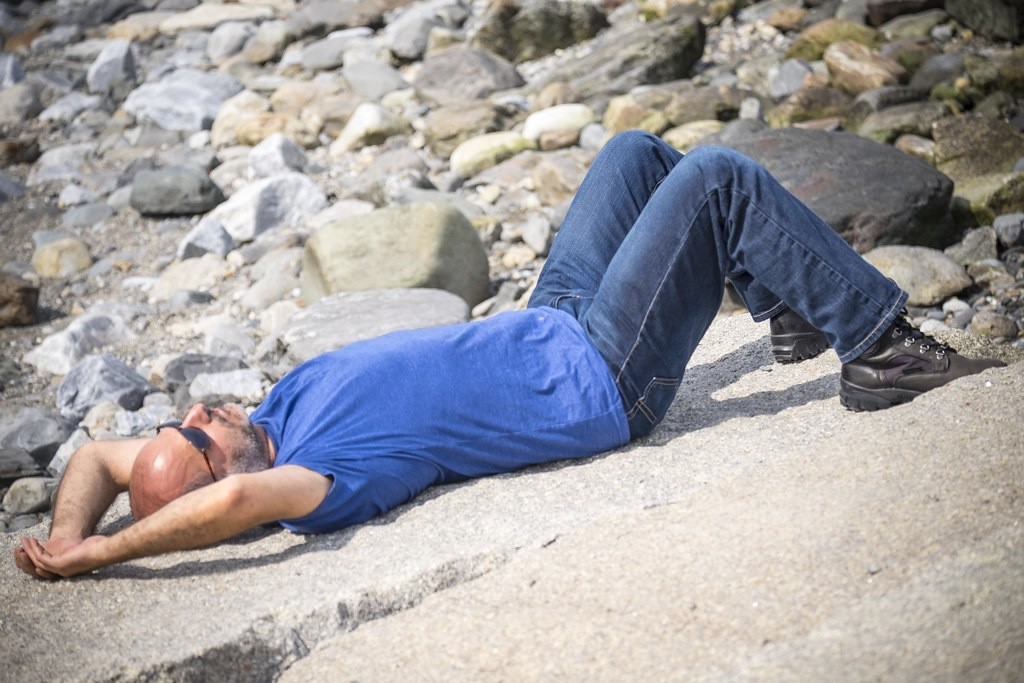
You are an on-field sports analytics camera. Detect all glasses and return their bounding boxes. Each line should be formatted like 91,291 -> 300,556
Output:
155,420 -> 217,483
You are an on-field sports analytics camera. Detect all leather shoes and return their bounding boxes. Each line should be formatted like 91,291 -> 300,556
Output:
770,308 -> 829,361
839,306 -> 1007,412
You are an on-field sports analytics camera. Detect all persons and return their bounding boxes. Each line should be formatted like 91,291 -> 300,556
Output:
15,129 -> 1008,578
